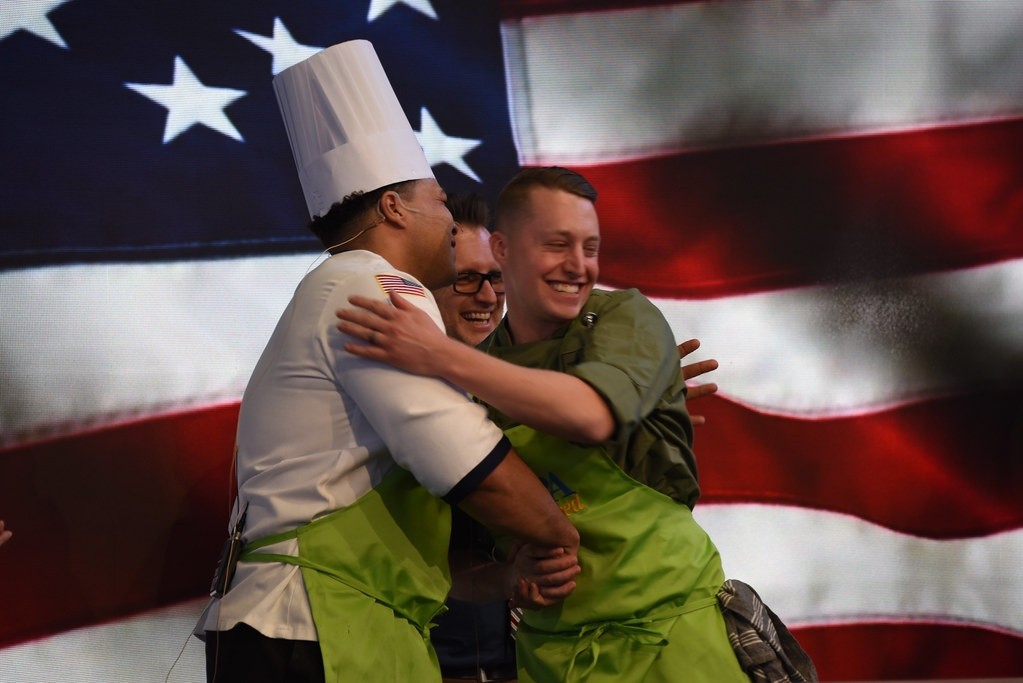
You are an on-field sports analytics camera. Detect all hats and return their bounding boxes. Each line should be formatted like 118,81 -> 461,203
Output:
273,40 -> 436,223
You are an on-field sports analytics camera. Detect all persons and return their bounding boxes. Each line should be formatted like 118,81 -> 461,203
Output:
337,164 -> 754,683
195,180 -> 579,683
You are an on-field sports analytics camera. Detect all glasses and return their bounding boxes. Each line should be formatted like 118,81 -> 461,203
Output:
453,269 -> 505,296
